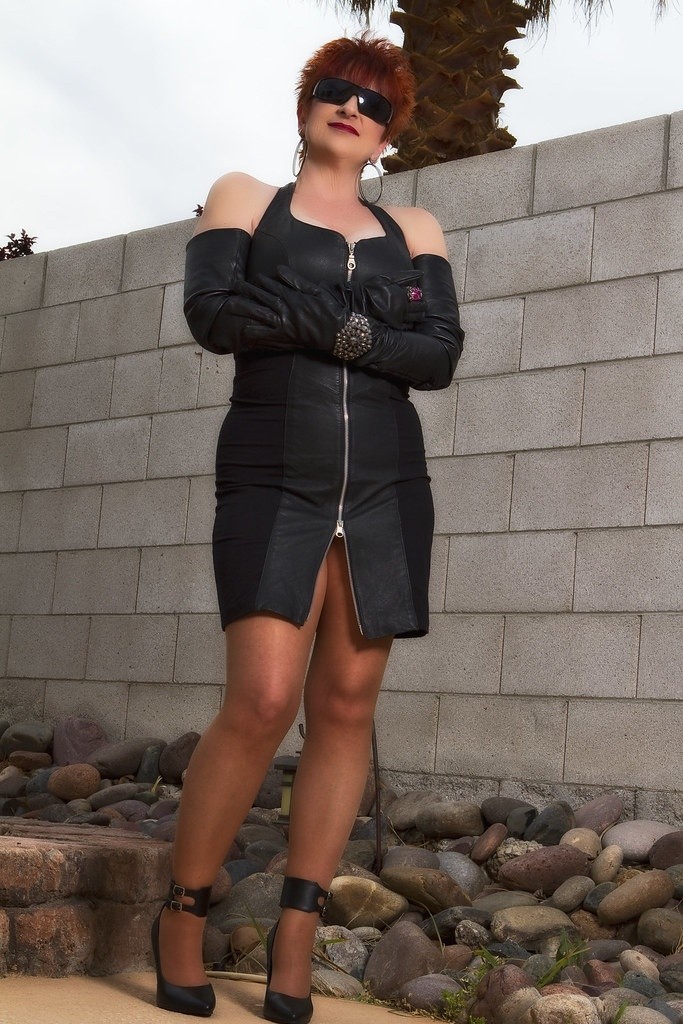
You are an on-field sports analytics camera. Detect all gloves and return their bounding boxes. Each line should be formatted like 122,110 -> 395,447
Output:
224,266 -> 451,391
321,270 -> 430,329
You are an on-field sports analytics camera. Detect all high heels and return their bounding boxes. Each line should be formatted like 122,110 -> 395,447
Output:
264,920 -> 314,1024
150,902 -> 216,1016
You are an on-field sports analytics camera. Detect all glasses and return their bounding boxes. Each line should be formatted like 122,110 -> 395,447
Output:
308,77 -> 394,125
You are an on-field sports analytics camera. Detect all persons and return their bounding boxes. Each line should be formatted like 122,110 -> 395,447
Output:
152,32 -> 466,1024
